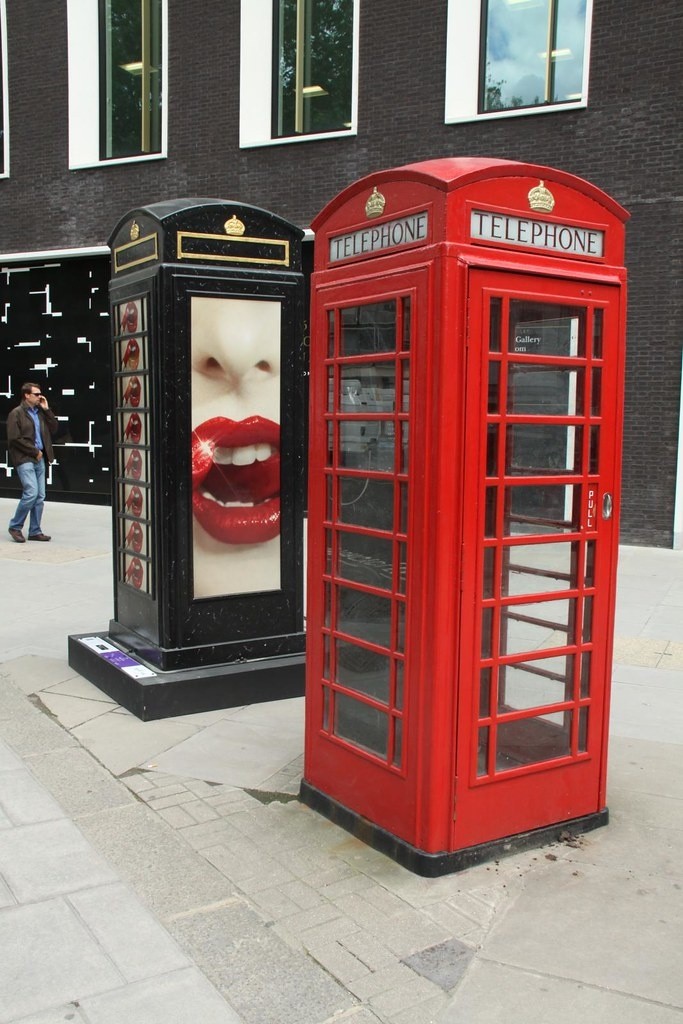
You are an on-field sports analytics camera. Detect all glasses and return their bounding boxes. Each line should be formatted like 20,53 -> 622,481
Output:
30,393 -> 43,396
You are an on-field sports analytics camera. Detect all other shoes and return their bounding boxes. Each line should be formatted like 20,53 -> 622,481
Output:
9,528 -> 25,542
28,534 -> 51,541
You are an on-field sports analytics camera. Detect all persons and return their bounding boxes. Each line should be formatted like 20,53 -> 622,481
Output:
120,301 -> 145,590
7,383 -> 58,542
191,298 -> 282,598
